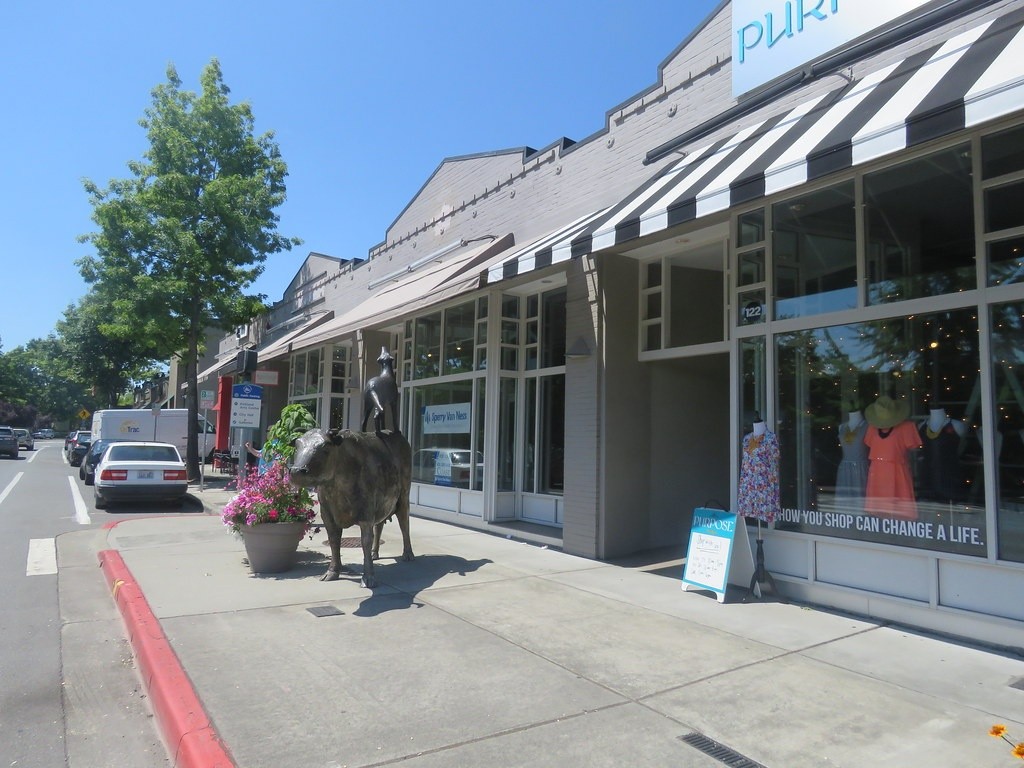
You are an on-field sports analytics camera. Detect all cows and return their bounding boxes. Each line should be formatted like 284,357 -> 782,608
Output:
287,426 -> 414,587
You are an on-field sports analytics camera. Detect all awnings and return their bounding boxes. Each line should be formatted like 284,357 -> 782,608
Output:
476,6 -> 1024,285
139,395 -> 174,409
181,233 -> 560,390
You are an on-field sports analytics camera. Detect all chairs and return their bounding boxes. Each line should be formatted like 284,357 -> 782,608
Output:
152,452 -> 164,459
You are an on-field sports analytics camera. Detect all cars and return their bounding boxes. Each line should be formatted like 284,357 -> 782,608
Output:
414,446 -> 483,488
80,439 -> 137,485
65,430 -> 91,467
94,441 -> 188,509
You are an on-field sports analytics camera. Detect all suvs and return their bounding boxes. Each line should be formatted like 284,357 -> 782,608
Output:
13,429 -> 34,451
0,426 -> 19,458
33,429 -> 55,439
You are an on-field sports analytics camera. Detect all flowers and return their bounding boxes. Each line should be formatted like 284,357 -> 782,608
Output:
222,404 -> 321,541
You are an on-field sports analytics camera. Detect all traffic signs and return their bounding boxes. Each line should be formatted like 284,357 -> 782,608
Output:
230,383 -> 263,428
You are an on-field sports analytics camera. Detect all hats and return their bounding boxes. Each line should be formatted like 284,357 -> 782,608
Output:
864,396 -> 910,430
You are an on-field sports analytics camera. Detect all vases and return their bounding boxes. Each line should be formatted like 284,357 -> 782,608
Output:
238,522 -> 306,572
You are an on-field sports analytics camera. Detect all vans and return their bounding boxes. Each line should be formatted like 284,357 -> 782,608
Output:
91,409 -> 230,464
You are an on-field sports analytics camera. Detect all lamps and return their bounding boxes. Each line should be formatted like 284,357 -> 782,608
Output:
266,313 -> 307,336
367,239 -> 468,290
214,345 -> 240,359
644,0 -> 997,164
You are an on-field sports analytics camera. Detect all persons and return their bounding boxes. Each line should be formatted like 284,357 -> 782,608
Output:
834,410 -> 971,523
736,420 -> 781,522
246,425 -> 282,478
